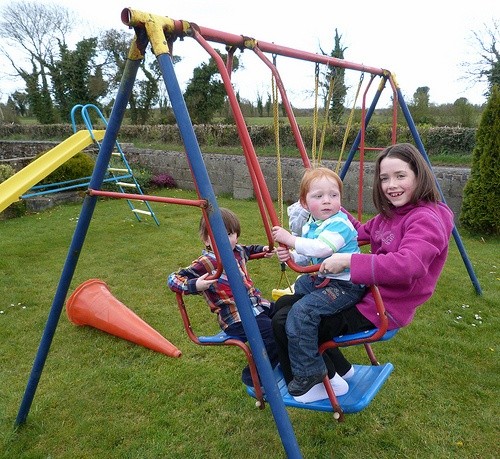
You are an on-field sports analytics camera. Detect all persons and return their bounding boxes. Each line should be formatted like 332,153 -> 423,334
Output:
271,143 -> 455,404
271,166 -> 368,396
167,207 -> 278,396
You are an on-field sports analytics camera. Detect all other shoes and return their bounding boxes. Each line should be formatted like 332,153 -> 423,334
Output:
241,365 -> 262,388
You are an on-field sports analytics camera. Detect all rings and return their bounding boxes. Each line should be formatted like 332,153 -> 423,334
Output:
323,268 -> 329,274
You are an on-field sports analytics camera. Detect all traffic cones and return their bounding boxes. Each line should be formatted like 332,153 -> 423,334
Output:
65,278 -> 183,359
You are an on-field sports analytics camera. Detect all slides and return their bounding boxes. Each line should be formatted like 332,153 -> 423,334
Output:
0,128 -> 106,212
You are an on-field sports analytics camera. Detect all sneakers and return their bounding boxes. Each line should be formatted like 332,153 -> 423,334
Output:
288,367 -> 328,396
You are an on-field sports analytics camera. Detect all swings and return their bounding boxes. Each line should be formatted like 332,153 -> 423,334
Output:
172,24 -> 398,416
